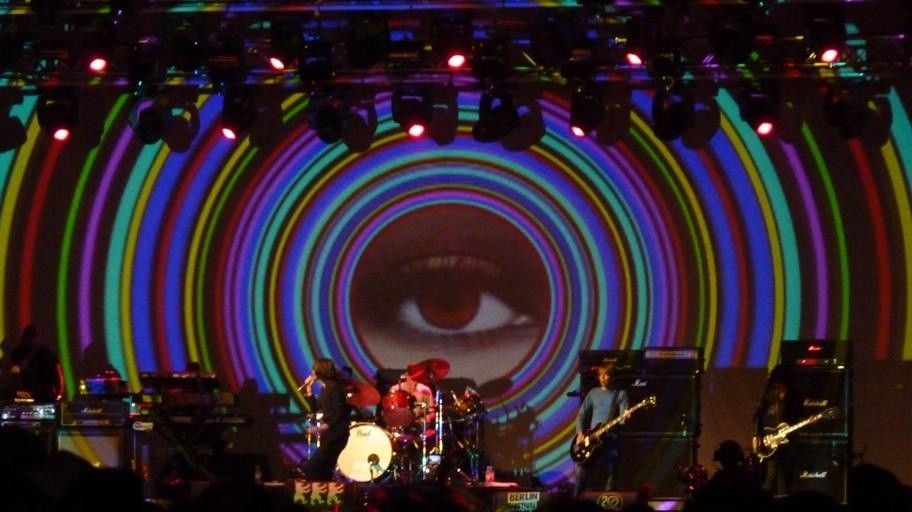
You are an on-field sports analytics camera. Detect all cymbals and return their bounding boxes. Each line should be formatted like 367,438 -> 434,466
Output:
340,381 -> 384,406
405,358 -> 451,384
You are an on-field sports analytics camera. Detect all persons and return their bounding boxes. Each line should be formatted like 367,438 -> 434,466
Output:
0,427 -> 910,512
9,324 -> 66,452
300,357 -> 350,483
746,379 -> 821,497
80,337 -> 120,381
389,361 -> 437,434
176,363 -> 226,482
574,360 -> 632,493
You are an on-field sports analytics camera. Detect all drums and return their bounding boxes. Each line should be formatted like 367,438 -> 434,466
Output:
453,390 -> 484,423
337,423 -> 398,483
380,389 -> 417,427
417,453 -> 449,480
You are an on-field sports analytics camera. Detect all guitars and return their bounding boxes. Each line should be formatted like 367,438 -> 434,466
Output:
753,406 -> 837,459
570,394 -> 657,466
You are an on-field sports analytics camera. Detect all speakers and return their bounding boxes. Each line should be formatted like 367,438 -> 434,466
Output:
580,370 -> 701,496
58,427 -> 124,468
773,372 -> 855,506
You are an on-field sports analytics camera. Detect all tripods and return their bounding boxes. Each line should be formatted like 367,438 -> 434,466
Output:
402,402 -> 483,485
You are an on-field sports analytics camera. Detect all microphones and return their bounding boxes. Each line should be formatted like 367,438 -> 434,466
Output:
398,374 -> 406,391
297,375 -> 312,392
368,454 -> 379,483
